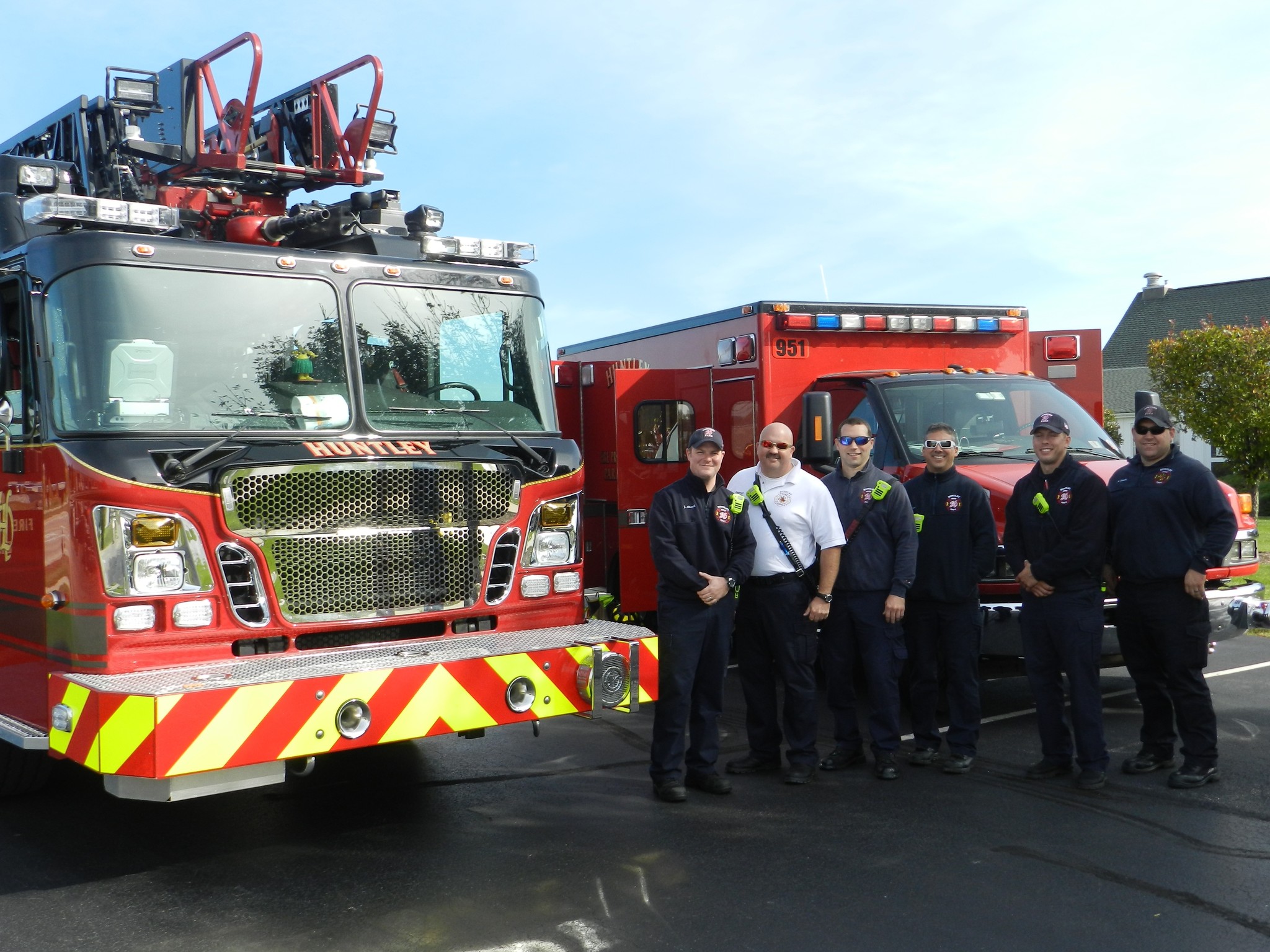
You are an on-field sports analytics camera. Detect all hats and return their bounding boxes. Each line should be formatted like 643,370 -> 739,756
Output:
1135,404 -> 1172,429
1030,412 -> 1070,436
688,428 -> 723,451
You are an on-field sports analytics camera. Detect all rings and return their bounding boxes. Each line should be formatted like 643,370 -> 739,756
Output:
709,597 -> 712,601
1194,589 -> 1199,593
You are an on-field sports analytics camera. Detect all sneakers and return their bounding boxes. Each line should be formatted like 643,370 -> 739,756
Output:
907,747 -> 939,765
1122,750 -> 1175,772
653,778 -> 686,800
820,746 -> 865,769
875,751 -> 899,778
726,751 -> 782,773
1027,759 -> 1071,779
684,770 -> 732,793
1076,768 -> 1108,789
1168,761 -> 1221,788
785,762 -> 814,784
942,754 -> 973,773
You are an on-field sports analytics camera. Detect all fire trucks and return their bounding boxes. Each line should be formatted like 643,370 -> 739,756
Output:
1,30 -> 660,803
556,302 -> 1264,657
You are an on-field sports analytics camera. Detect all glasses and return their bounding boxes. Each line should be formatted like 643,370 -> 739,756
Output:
924,440 -> 955,448
759,440 -> 791,450
838,436 -> 870,445
1135,426 -> 1165,435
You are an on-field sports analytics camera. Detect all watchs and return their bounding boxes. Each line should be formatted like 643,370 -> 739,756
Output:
727,576 -> 735,588
816,592 -> 833,603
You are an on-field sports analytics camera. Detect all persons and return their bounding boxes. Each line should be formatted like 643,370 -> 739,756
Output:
813,416 -> 920,781
1002,412 -> 1109,789
1105,405 -> 1238,787
902,423 -> 998,773
726,422 -> 847,783
647,428 -> 758,802
